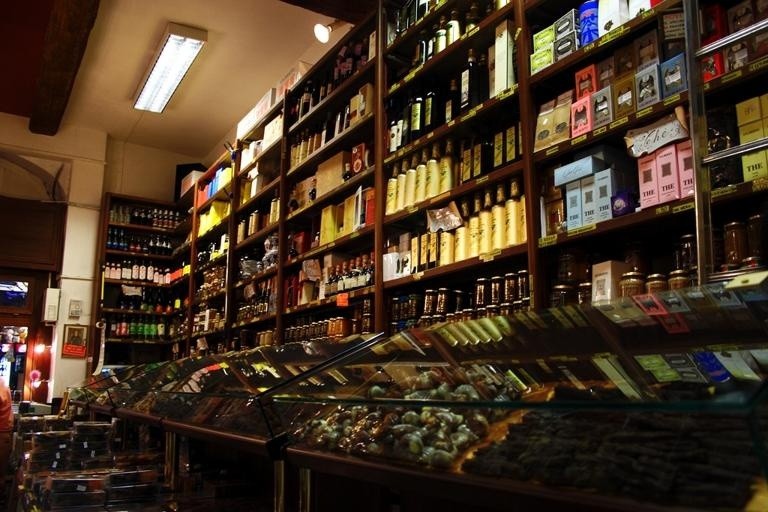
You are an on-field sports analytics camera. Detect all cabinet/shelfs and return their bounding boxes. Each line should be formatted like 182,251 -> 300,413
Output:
86,1 -> 765,510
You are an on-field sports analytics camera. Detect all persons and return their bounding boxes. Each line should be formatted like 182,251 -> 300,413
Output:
0,378 -> 15,511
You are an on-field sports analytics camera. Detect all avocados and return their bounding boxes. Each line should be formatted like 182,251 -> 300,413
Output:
310,405 -> 516,468
368,365 -> 503,401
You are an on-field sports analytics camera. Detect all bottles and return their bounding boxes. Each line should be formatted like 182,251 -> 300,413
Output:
269,187 -> 280,224
328,34 -> 369,83
724,211 -> 768,263
386,101 -> 523,217
109,204 -> 183,230
109,318 -> 188,339
411,178 -> 527,276
325,252 -> 374,296
288,164 -> 351,213
105,259 -> 191,283
413,0 -> 492,73
289,79 -> 333,128
118,287 -> 188,314
383,47 -> 488,151
290,98 -> 350,172
107,229 -> 172,257
237,279 -> 272,322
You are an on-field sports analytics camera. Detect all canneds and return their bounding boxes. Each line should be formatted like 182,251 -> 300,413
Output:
231,328 -> 273,352
390,269 -> 530,336
549,212 -> 768,310
283,296 -> 374,343
